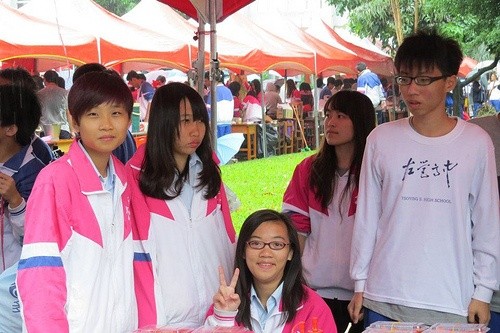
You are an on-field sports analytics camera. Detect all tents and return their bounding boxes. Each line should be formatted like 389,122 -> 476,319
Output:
458,56 -> 500,81
0,0 -> 392,157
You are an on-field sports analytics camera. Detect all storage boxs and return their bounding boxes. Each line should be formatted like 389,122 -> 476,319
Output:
277,104 -> 293,119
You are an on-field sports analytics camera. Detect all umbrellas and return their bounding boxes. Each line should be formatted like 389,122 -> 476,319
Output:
217,133 -> 245,167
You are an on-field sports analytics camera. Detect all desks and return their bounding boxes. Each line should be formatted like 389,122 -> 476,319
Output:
47,139 -> 73,154
229,117 -> 325,160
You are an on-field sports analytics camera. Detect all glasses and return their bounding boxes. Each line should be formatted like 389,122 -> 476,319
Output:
247,241 -> 290,250
394,75 -> 447,86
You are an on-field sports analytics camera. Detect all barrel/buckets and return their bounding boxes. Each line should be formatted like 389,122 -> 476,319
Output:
43,124 -> 61,140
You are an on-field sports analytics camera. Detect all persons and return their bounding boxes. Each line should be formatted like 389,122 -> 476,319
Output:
122,62 -> 466,160
0,68 -> 53,274
473,75 -> 482,116
204,209 -> 337,333
126,82 -> 237,333
0,69 -> 160,333
35,70 -> 76,140
280,90 -> 376,333
347,28 -> 500,330
488,72 -> 500,113
